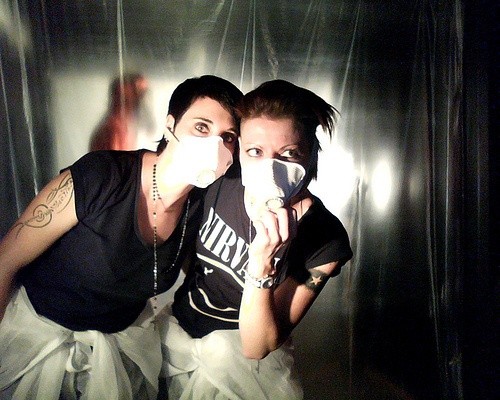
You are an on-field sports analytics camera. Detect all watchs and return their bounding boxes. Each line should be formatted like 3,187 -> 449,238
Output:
244,271 -> 274,289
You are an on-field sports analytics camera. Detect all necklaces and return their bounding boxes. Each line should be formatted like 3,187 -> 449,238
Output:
250,194 -> 303,280
153,156 -> 191,311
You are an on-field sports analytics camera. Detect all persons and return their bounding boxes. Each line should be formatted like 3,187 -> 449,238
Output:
0,74 -> 243,400
155,78 -> 354,399
89,75 -> 150,154
